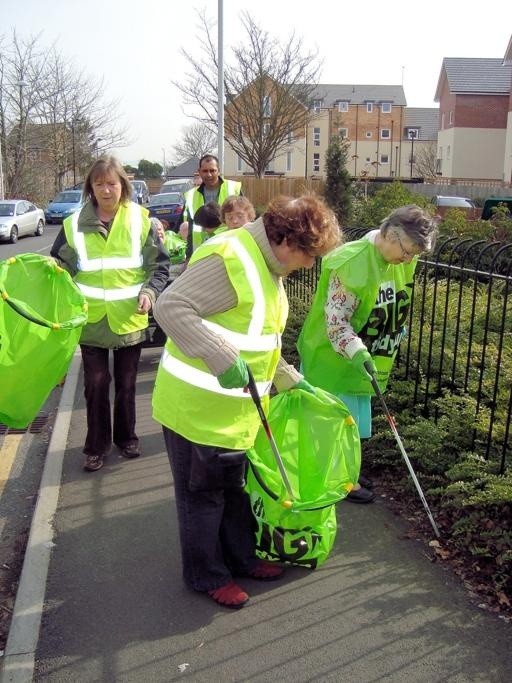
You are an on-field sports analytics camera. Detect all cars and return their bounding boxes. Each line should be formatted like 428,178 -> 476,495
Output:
44,189 -> 85,224
0,199 -> 46,243
429,195 -> 476,223
128,178 -> 195,226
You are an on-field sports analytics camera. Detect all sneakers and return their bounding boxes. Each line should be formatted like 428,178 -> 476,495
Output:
246,562 -> 285,583
83,445 -> 112,470
345,487 -> 375,504
358,474 -> 371,486
207,579 -> 248,608
120,439 -> 141,457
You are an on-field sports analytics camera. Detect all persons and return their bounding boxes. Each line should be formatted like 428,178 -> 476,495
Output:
216,195 -> 257,229
296,201 -> 443,503
178,151 -> 248,260
50,153 -> 169,471
150,192 -> 341,608
160,221 -> 190,265
150,211 -> 166,248
189,198 -> 226,242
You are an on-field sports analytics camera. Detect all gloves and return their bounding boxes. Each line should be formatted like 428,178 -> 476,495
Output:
352,350 -> 377,383
217,355 -> 248,389
292,380 -> 317,395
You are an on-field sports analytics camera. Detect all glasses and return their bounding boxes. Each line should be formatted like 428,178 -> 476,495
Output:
394,230 -> 417,259
